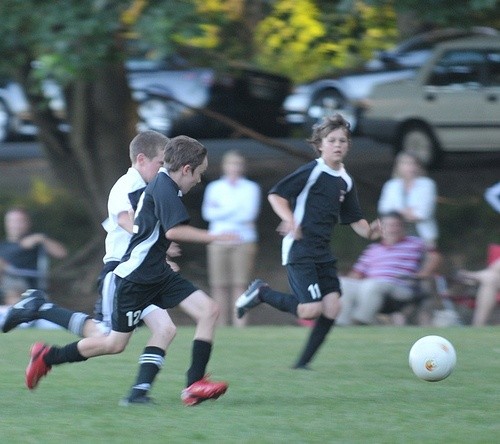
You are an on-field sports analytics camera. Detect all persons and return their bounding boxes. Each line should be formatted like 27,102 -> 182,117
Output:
457,257 -> 500,327
376,150 -> 440,325
333,211 -> 443,325
0,207 -> 68,304
201,149 -> 261,329
234,114 -> 383,372
0,130 -> 182,408
25,135 -> 240,407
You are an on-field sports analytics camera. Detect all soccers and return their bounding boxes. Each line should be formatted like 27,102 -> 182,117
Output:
407,334 -> 459,382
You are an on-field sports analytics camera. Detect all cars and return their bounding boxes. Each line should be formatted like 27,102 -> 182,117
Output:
283,26 -> 500,171
0,39 -> 293,145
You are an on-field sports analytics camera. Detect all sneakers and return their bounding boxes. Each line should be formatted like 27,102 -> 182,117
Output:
3,289 -> 46,333
120,396 -> 155,406
181,378 -> 227,405
25,343 -> 51,388
236,279 -> 271,318
